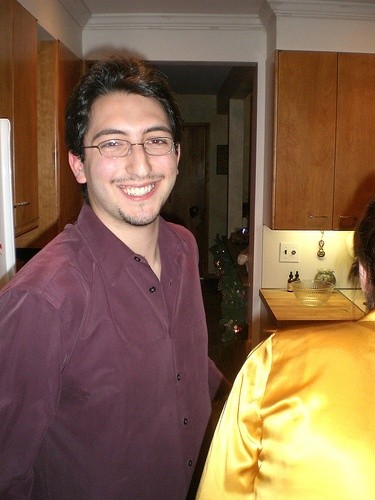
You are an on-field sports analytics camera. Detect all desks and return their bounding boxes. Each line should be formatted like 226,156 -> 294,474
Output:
258,287 -> 369,342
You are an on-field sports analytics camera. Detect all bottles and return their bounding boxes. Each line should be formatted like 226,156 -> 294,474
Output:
287,271 -> 300,292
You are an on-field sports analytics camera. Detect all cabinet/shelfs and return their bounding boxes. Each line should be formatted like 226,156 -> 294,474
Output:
263,49 -> 375,231
0,2 -> 39,234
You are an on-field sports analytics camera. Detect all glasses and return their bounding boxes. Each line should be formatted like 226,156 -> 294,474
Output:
80,137 -> 176,159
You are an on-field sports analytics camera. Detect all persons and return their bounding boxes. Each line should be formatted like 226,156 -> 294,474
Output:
0,55 -> 235,500
192,196 -> 375,499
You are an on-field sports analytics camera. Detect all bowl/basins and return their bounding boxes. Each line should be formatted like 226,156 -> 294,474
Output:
292,280 -> 334,306
